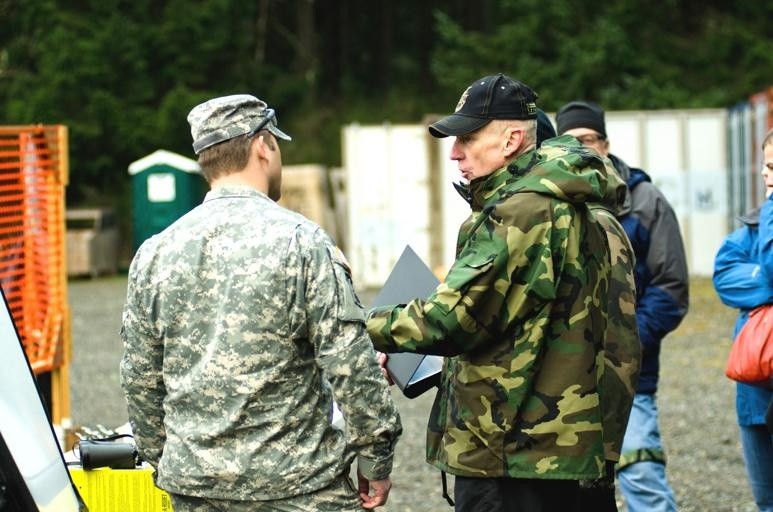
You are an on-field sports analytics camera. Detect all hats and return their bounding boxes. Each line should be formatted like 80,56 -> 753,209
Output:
555,100 -> 606,135
427,72 -> 539,140
186,93 -> 292,156
535,107 -> 555,149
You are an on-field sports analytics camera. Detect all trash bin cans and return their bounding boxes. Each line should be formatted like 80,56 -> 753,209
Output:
126,147 -> 209,263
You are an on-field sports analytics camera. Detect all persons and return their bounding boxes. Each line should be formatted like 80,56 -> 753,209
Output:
555,102 -> 688,512
363,74 -> 643,512
120,94 -> 403,512
711,128 -> 773,512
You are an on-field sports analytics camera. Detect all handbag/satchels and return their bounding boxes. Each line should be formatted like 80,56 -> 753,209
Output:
724,304 -> 772,388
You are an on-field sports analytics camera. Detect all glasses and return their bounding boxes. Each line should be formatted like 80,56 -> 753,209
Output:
575,135 -> 605,145
